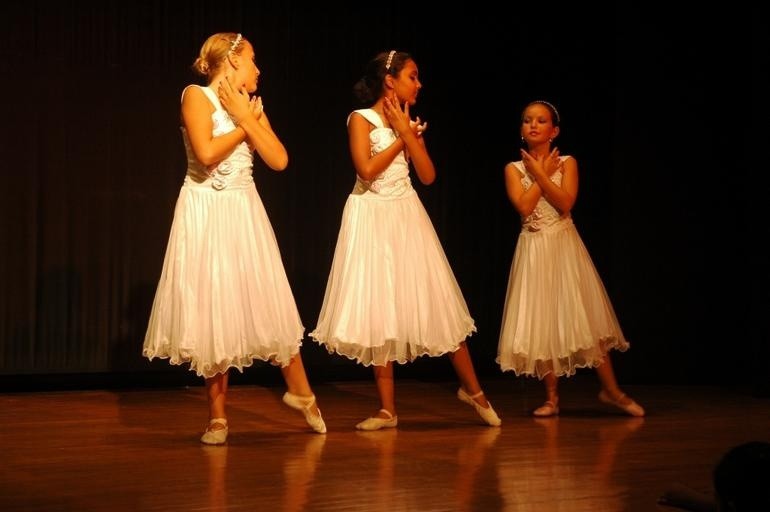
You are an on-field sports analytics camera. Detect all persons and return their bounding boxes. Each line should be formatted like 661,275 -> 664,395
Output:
355,427 -> 503,511
494,99 -> 647,418
142,32 -> 327,446
308,50 -> 502,433
202,432 -> 327,512
493,417 -> 647,512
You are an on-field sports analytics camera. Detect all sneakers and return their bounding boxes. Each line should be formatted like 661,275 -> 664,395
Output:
283,393 -> 326,433
356,410 -> 398,431
598,390 -> 645,416
531,401 -> 560,417
456,388 -> 502,426
201,419 -> 229,445
